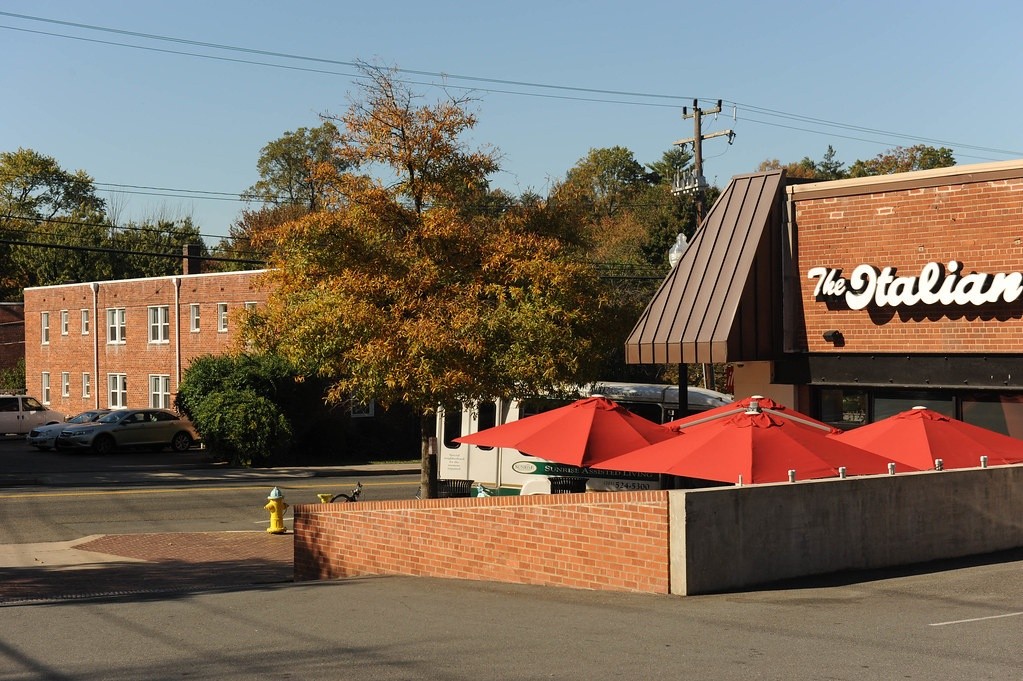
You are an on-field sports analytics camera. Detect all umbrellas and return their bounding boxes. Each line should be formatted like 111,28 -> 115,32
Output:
450,394 -> 1023,486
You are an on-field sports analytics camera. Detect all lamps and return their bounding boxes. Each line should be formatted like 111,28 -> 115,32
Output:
823,330 -> 844,346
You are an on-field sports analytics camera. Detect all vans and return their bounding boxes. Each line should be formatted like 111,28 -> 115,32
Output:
437,376 -> 734,502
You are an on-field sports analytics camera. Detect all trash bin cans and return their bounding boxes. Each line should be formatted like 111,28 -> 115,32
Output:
436,479 -> 474,498
548,476 -> 590,494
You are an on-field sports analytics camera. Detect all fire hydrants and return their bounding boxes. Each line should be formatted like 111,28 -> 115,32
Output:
263,486 -> 289,534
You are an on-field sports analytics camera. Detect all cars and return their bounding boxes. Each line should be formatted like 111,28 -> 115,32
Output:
59,408 -> 202,456
0,393 -> 65,438
26,408 -> 115,454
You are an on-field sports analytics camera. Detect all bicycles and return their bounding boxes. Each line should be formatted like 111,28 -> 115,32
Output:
328,479 -> 364,503
